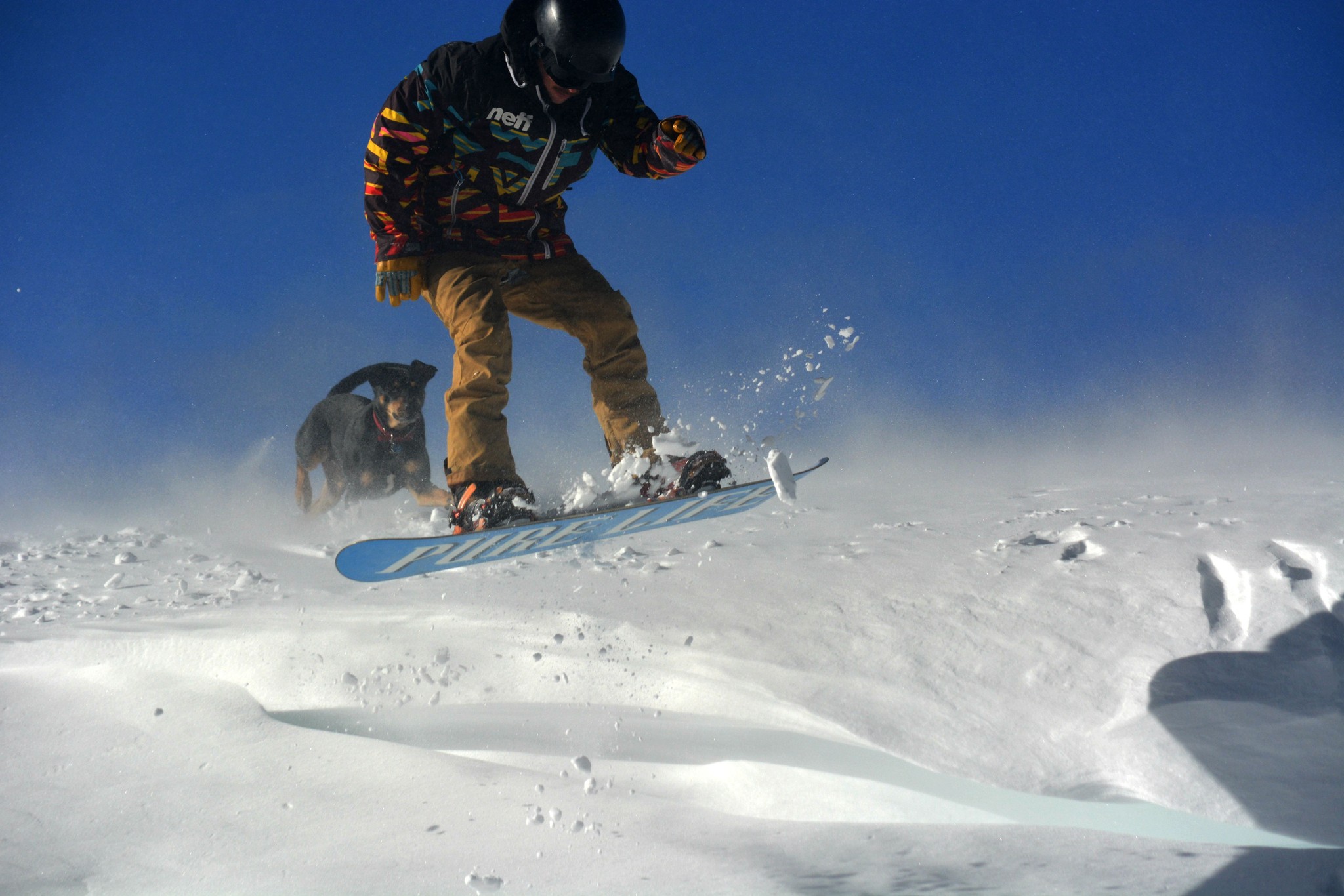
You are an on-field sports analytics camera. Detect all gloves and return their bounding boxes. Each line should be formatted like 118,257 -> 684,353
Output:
662,119 -> 706,161
375,257 -> 429,307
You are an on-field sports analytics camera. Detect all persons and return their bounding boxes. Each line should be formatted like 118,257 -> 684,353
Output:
363,0 -> 732,534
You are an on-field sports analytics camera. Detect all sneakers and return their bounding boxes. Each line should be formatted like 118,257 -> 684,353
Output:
635,450 -> 731,502
442,458 -> 534,531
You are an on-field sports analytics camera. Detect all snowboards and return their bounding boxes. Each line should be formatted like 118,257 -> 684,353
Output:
335,455 -> 831,583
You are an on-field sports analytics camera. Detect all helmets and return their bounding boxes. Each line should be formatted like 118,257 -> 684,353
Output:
528,0 -> 626,90
